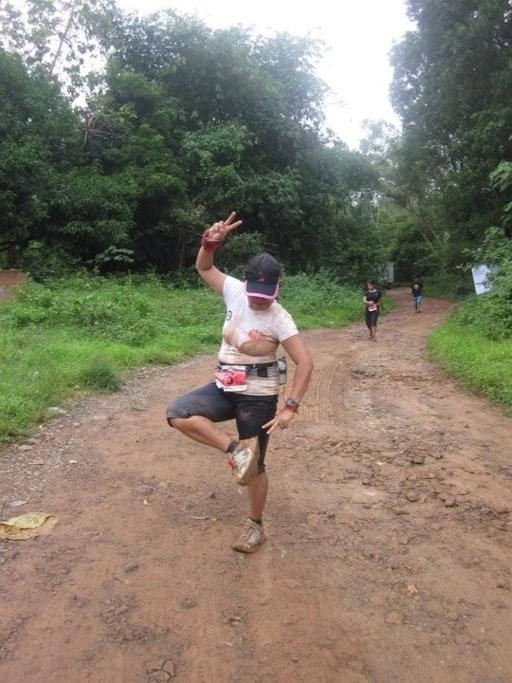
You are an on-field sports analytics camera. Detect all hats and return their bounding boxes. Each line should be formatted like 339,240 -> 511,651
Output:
245,253 -> 282,300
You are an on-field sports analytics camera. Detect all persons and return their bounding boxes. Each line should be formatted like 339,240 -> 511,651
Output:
408,275 -> 424,314
162,208 -> 316,554
362,278 -> 382,342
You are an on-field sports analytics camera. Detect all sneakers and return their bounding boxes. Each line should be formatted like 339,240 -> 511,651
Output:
230,435 -> 262,486
233,517 -> 265,554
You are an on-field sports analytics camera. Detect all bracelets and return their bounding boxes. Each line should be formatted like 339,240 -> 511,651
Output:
201,228 -> 224,253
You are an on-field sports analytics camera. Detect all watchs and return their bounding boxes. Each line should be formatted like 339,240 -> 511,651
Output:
285,397 -> 299,410
